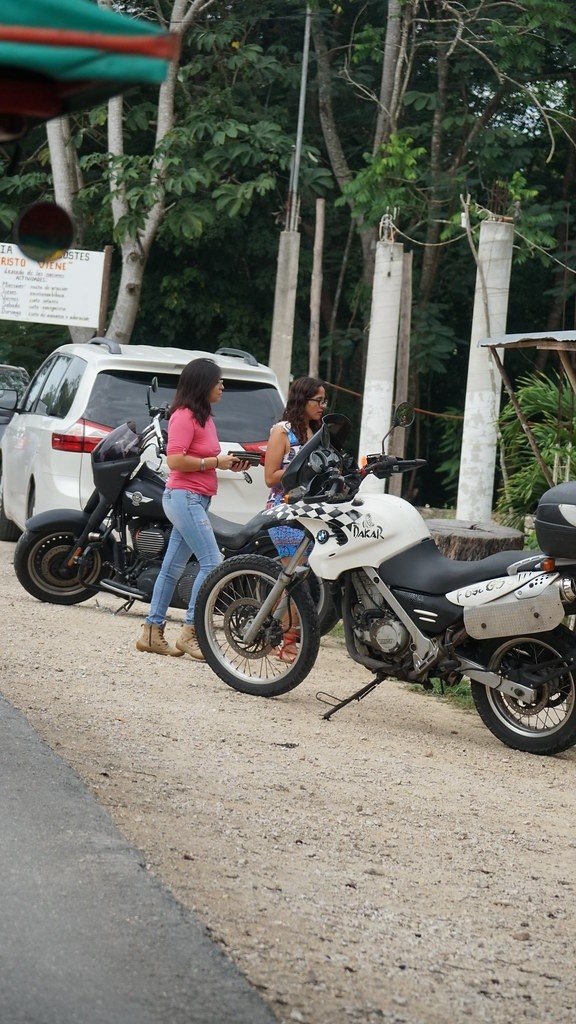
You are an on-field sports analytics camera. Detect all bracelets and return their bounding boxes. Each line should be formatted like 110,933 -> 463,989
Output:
201,458 -> 205,471
215,456 -> 218,468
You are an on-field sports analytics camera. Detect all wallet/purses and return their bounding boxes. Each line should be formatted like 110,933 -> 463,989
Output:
228,451 -> 262,467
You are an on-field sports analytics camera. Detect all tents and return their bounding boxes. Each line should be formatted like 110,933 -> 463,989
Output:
0,0 -> 178,142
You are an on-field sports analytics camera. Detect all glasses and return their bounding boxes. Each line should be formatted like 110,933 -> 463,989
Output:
308,399 -> 328,406
217,380 -> 223,386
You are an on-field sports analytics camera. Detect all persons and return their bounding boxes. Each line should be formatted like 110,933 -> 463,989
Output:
136,358 -> 253,662
265,378 -> 327,664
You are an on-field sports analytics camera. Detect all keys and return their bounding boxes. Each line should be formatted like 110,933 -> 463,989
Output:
243,471 -> 252,484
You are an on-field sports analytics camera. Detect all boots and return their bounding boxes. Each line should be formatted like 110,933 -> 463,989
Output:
136,621 -> 186,657
176,625 -> 204,660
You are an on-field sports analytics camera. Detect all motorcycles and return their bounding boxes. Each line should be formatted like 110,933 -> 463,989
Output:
14,377 -> 342,647
194,401 -> 576,755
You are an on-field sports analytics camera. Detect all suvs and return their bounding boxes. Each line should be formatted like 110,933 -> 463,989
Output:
0,338 -> 300,542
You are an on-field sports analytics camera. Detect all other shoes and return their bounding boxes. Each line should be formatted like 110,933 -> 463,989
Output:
274,656 -> 294,664
